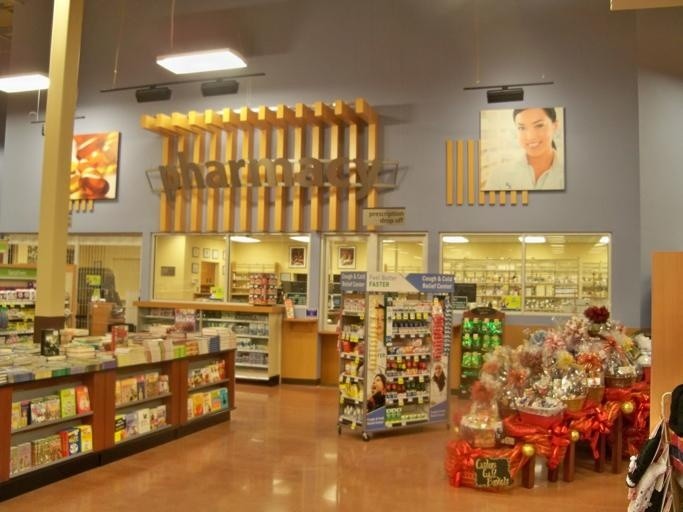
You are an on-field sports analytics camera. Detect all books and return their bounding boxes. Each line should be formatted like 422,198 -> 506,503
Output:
0,308 -> 237,479
0,238 -> 74,321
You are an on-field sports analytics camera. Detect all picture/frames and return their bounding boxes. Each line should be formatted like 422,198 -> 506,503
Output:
192,247 -> 227,275
338,246 -> 356,269
289,247 -> 306,268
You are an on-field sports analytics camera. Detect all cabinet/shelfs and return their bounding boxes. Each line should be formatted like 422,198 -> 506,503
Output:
0,346 -> 236,501
443,256 -> 608,311
231,261 -> 279,302
134,300 -> 285,384
0,265 -> 78,346
459,306 -> 505,399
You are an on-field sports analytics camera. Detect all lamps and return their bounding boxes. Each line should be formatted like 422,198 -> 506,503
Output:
100,72 -> 265,102
462,81 -> 554,102
154,0 -> 248,76
0,72 -> 51,94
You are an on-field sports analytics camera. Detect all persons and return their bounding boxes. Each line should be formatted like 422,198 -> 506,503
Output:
365,374 -> 386,414
430,363 -> 447,406
508,107 -> 564,190
